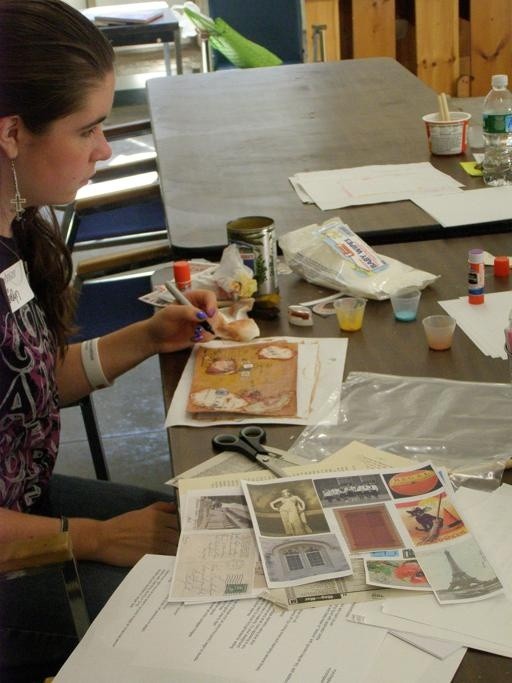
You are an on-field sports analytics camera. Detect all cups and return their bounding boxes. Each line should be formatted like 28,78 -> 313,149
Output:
332,297 -> 366,331
419,314 -> 456,351
389,286 -> 423,322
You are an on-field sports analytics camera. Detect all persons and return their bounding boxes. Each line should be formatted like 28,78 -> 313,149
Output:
270,485 -> 310,539
0,0 -> 225,683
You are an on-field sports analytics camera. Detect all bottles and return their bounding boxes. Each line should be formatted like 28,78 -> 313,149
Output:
227,215 -> 280,299
482,75 -> 512,186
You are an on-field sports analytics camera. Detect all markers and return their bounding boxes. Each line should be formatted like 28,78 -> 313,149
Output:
163,280 -> 216,335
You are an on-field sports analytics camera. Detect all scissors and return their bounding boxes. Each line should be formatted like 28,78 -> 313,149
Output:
212,426 -> 288,478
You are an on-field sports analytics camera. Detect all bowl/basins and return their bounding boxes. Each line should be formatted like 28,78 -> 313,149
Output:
422,110 -> 474,157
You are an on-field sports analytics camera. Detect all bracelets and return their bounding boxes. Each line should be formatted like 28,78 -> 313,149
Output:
81,338 -> 113,391
60,517 -> 72,539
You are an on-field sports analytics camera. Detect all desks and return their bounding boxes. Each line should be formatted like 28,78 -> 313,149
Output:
144,56 -> 512,257
151,230 -> 512,683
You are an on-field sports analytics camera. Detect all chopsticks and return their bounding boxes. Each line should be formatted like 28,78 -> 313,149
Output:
436,94 -> 452,123
